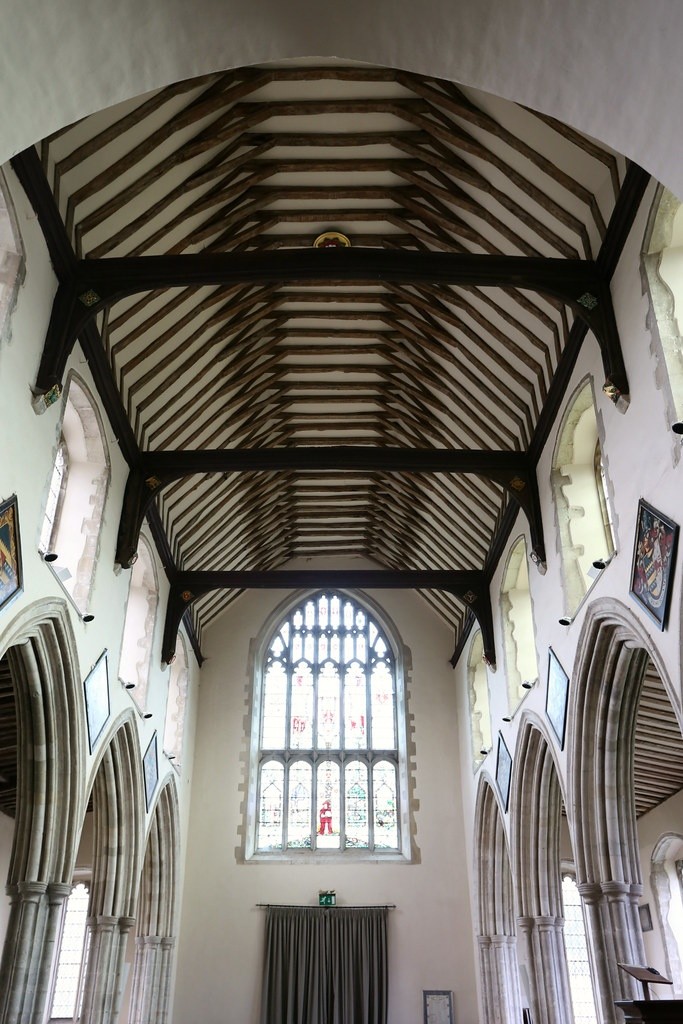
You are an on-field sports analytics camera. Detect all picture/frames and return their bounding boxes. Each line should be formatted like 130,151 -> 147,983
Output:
629,498 -> 680,632
142,729 -> 160,813
492,730 -> 513,814
544,646 -> 570,752
423,990 -> 455,1024
83,649 -> 111,755
638,903 -> 653,932
0,494 -> 24,613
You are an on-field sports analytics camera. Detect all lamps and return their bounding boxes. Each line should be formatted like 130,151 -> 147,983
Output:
38,549 -> 95,626
117,677 -> 152,721
501,679 -> 538,722
559,549 -> 614,625
